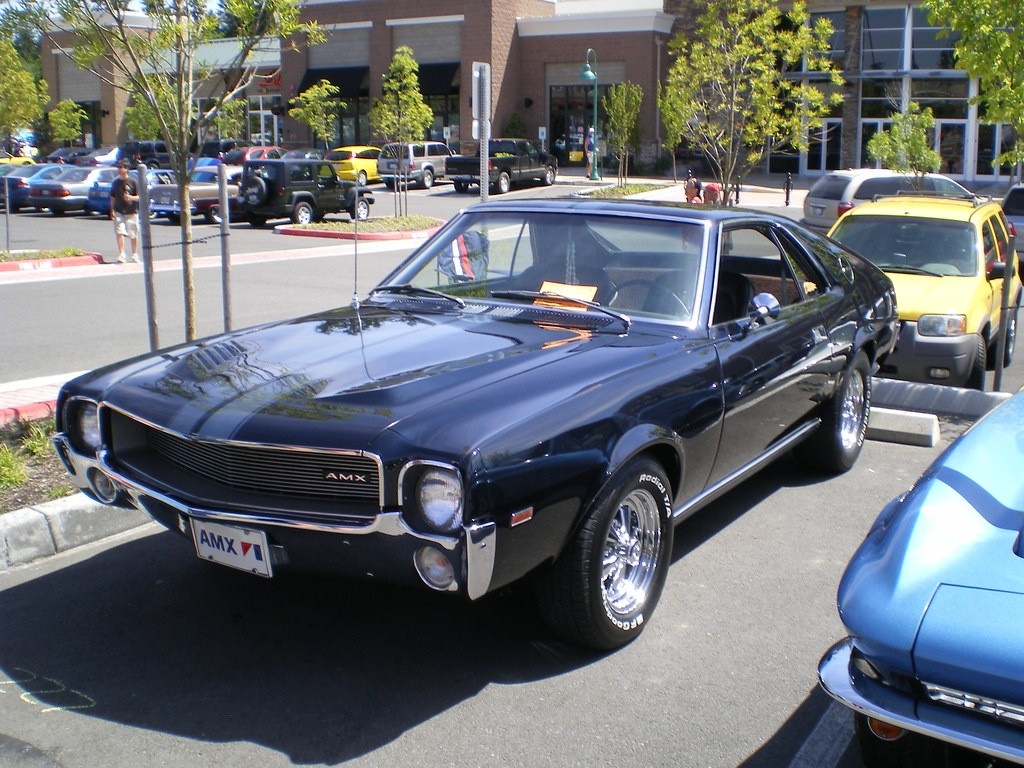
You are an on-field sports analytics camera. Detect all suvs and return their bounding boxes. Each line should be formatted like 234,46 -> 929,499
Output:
823,192 -> 1020,390
376,141 -> 463,190
238,159 -> 373,227
120,140 -> 194,169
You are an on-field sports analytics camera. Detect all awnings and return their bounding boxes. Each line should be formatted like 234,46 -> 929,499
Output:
382,61 -> 461,96
298,65 -> 370,98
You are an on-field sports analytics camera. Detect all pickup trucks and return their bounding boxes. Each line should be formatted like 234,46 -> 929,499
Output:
444,137 -> 559,194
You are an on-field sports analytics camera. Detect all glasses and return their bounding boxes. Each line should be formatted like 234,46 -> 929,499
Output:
117,165 -> 123,168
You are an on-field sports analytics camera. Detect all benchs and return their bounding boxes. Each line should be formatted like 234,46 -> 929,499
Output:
603,265 -> 816,315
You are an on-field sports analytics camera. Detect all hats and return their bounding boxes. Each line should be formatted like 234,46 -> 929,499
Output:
706,184 -> 721,193
589,128 -> 594,132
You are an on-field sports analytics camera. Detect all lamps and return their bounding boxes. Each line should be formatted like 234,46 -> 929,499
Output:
101,109 -> 109,118
844,80 -> 855,99
271,104 -> 285,117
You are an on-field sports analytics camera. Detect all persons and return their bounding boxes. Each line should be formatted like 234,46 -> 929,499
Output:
585,128 -> 600,178
682,177 -> 733,255
111,161 -> 139,263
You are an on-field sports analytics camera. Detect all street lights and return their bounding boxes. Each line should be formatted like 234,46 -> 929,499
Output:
580,48 -> 600,180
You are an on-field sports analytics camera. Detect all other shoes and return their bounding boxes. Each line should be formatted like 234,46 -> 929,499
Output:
116,253 -> 125,261
131,254 -> 139,264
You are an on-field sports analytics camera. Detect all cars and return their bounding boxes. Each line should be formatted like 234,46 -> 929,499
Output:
223,146 -> 295,165
320,145 -> 383,187
1002,181 -> 1024,262
0,148 -> 95,213
73,146 -> 120,167
200,140 -> 257,158
815,380 -> 1024,764
281,148 -> 326,181
53,198 -> 897,650
146,165 -> 242,224
27,167 -> 119,217
800,166 -> 988,233
88,169 -> 177,220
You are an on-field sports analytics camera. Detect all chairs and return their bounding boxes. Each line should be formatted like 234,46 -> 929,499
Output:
643,270 -> 759,325
503,264 -> 610,306
